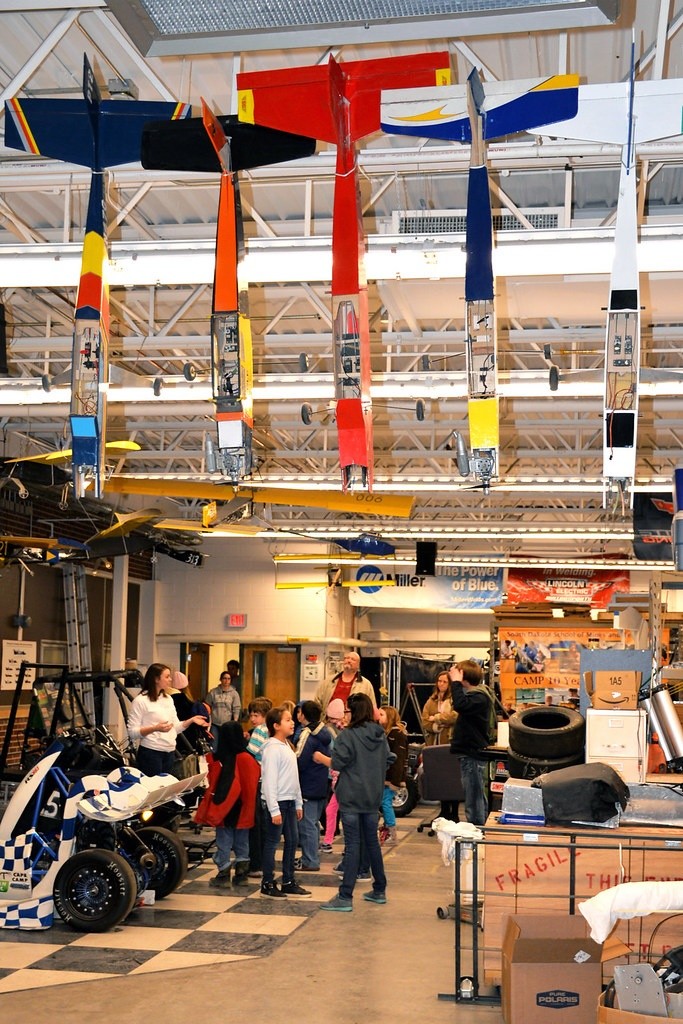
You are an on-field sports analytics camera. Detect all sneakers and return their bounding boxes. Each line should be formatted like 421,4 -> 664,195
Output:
282,878 -> 311,898
318,842 -> 333,853
319,893 -> 352,911
338,870 -> 372,882
363,889 -> 386,903
261,882 -> 287,901
333,862 -> 344,875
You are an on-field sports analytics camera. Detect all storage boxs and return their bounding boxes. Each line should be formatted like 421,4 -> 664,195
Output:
501,912 -> 602,1024
584,671 -> 642,710
597,987 -> 682,1024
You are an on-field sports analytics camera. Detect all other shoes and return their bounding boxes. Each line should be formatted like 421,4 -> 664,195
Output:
293,857 -> 320,871
247,864 -> 275,877
319,827 -> 340,837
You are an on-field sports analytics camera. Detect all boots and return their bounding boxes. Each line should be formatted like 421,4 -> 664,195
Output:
209,864 -> 231,887
232,861 -> 249,886
378,822 -> 398,846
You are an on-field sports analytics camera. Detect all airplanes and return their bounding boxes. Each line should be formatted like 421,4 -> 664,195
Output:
84,476 -> 417,536
138,97 -> 328,497
380,67 -> 579,496
272,552 -> 397,596
236,51 -> 452,493
0,441 -> 209,576
4,51 -> 192,498
524,25 -> 683,517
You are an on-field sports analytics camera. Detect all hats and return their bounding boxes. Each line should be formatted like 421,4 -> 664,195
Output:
327,699 -> 344,719
173,671 -> 188,689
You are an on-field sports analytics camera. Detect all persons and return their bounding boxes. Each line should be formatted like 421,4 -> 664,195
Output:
313,692 -> 397,911
545,695 -> 557,706
420,660 -> 498,840
246,696 -> 273,878
163,671 -> 210,818
192,720 -> 261,888
261,699 -> 332,900
127,662 -> 211,774
314,651 -> 410,853
515,641 -> 545,673
502,702 -> 516,720
568,688 -> 579,698
501,640 -> 517,659
204,671 -> 241,753
227,660 -> 241,696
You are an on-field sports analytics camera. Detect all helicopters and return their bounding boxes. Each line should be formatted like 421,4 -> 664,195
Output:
267,527 -> 395,556
